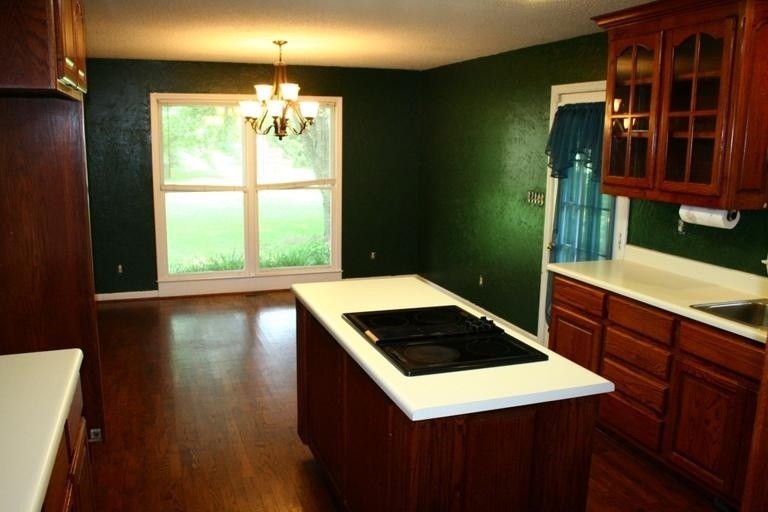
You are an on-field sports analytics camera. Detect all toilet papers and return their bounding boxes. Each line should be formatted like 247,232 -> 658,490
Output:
678,205 -> 740,229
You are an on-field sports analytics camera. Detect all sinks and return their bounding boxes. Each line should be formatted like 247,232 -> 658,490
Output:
690,298 -> 768,331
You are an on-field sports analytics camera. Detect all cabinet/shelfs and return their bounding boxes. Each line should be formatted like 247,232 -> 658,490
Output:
40,374 -> 96,510
597,293 -> 672,459
590,0 -> 768,214
549,271 -> 606,376
662,312 -> 768,511
2,0 -> 88,101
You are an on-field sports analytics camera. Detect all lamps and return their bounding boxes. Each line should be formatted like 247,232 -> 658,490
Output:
241,41 -> 319,142
